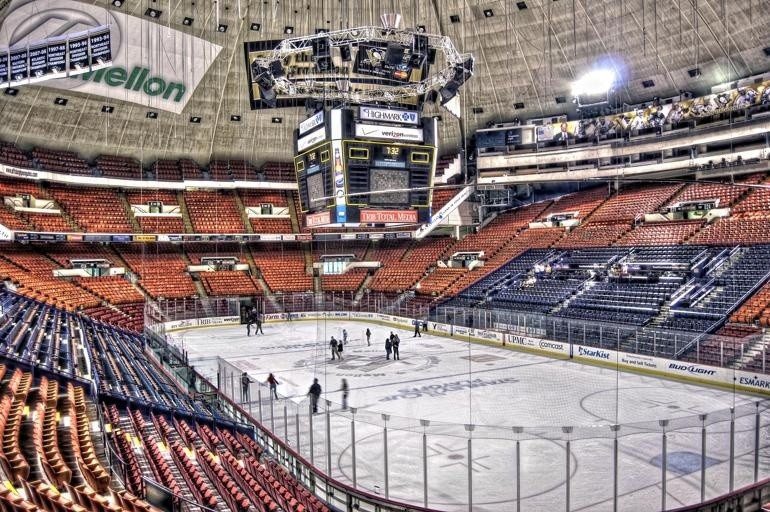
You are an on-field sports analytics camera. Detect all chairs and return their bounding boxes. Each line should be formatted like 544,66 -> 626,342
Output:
0,364 -> 331,512
428,186 -> 582,344
583,176 -> 766,371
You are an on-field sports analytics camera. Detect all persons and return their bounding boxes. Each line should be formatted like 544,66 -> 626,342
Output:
241,372 -> 255,404
328,336 -> 344,362
338,377 -> 349,410
286,310 -> 292,322
385,331 -> 400,361
554,83 -> 770,142
412,314 -> 428,338
365,327 -> 371,347
246,305 -> 263,336
307,378 -> 321,413
343,328 -> 349,345
264,373 -> 280,400
